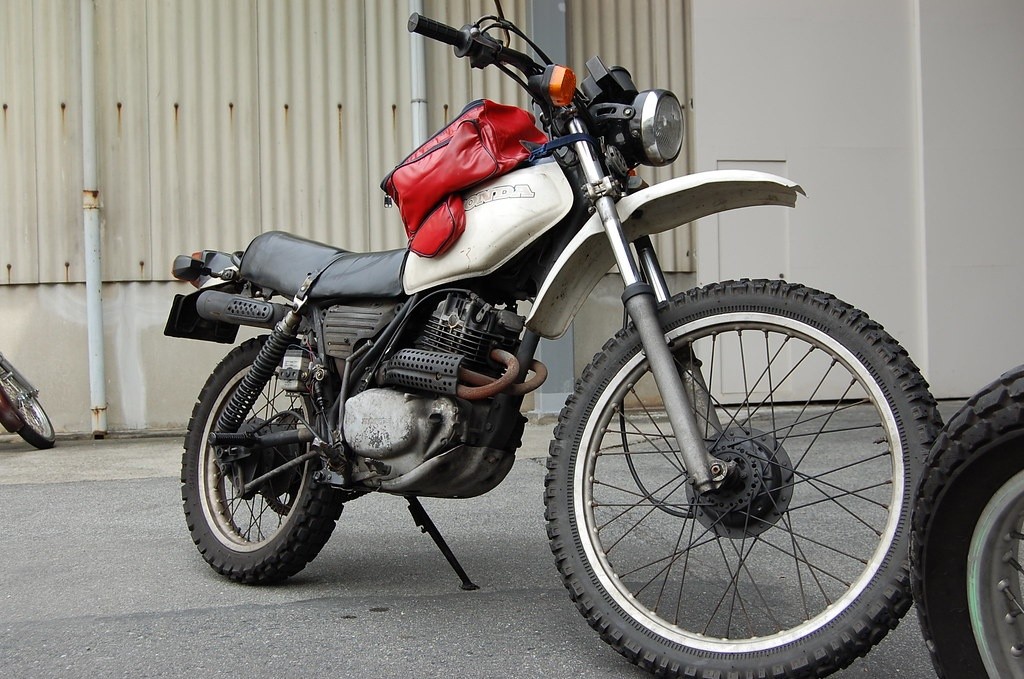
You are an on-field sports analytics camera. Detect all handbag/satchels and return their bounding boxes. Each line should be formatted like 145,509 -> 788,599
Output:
379,101 -> 598,259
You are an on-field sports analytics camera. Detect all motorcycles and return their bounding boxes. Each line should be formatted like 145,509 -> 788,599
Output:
0,350 -> 58,451
165,0 -> 955,679
